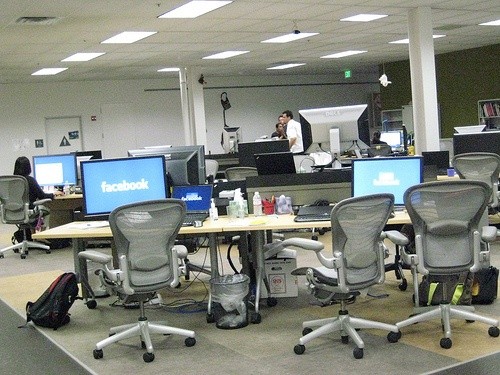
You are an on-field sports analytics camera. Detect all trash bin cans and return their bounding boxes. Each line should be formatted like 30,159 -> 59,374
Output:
209,274 -> 250,330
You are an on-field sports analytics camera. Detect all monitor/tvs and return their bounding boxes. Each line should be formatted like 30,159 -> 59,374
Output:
254,152 -> 297,175
32,145 -> 206,218
223,127 -> 242,153
380,131 -> 401,148
452,125 -> 500,158
298,104 -> 371,169
351,156 -> 424,206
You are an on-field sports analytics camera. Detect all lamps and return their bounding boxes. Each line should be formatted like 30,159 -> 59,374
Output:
379,59 -> 393,86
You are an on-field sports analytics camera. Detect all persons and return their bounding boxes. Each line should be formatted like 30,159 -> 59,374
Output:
270,110 -> 305,156
373,131 -> 386,143
13,156 -> 64,250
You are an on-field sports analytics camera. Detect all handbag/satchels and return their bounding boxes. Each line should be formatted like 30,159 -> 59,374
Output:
413,266 -> 499,306
310,284 -> 361,304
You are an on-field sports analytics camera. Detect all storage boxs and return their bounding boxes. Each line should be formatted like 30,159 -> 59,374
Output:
261,257 -> 299,298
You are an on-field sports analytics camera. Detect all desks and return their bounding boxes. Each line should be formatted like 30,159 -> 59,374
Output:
41,194 -> 83,211
32,217 -> 229,308
223,201 -> 500,314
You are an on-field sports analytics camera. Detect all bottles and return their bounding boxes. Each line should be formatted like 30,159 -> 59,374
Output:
253,192 -> 262,216
65,180 -> 69,195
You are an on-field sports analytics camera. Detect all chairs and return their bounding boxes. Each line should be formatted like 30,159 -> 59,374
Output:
282,193 -> 399,358
368,144 -> 394,156
0,175 -> 51,260
387,181 -> 500,349
225,166 -> 258,183
76,199 -> 196,361
452,153 -> 500,212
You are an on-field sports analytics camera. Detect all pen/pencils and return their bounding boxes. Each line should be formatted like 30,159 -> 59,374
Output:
272,196 -> 274,203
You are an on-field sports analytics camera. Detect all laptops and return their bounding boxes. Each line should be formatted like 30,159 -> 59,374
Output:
171,185 -> 214,224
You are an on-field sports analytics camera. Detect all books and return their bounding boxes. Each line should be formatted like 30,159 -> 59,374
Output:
480,101 -> 500,117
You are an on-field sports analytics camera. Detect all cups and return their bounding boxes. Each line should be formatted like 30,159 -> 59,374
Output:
447,169 -> 457,177
264,204 -> 275,215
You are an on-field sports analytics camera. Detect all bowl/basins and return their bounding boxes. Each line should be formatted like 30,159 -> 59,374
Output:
454,125 -> 487,133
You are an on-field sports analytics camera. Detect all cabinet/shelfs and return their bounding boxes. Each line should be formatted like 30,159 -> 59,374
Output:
381,109 -> 405,131
478,99 -> 500,131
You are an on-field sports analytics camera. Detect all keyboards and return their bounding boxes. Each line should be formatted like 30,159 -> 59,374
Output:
295,214 -> 331,222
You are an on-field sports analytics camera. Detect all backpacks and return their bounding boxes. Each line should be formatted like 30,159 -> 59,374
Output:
26,272 -> 79,331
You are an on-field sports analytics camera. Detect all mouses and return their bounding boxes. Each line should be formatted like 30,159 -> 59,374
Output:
194,220 -> 203,228
